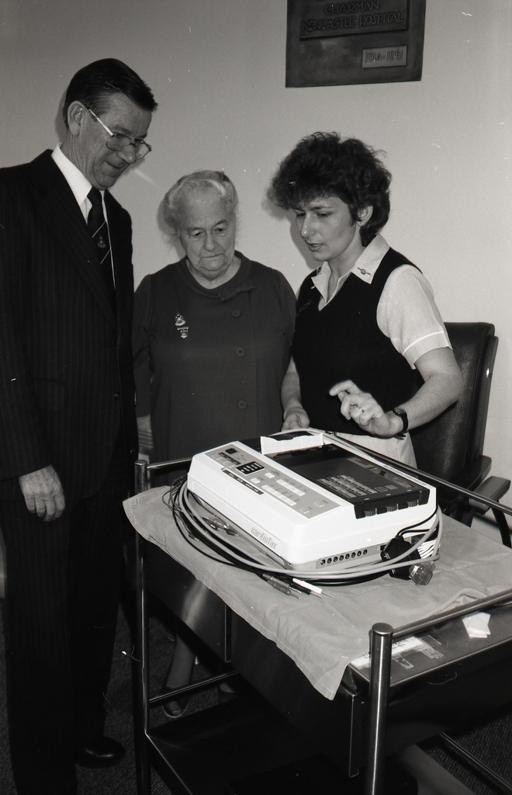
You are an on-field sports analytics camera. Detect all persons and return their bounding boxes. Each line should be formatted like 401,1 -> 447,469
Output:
131,170 -> 295,719
0,58 -> 156,794
261,130 -> 467,469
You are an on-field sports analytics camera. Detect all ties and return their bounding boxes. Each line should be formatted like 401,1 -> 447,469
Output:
87,186 -> 115,289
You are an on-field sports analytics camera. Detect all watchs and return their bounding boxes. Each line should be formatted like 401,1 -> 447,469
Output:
391,403 -> 411,441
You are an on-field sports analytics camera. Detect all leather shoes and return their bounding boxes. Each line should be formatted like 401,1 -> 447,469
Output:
162,687 -> 192,718
77,732 -> 123,766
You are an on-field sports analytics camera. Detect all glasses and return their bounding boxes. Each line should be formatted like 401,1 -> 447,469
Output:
87,105 -> 151,161
